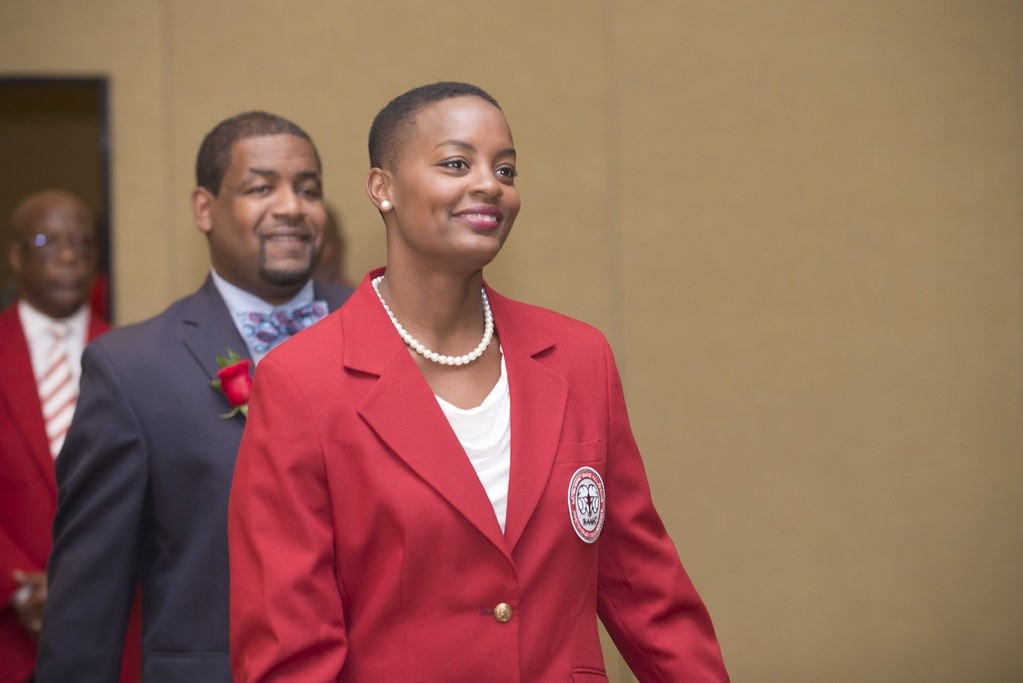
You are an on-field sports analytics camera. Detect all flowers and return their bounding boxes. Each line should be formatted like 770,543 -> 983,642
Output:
207,346 -> 254,419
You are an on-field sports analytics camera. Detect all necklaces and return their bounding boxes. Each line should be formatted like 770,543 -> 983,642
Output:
371,275 -> 494,366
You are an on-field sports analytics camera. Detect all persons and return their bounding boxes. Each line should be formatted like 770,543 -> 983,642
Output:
0,185 -> 141,682
227,82 -> 730,683
25,111 -> 359,683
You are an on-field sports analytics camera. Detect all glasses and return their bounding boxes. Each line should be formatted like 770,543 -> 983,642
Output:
14,232 -> 97,255
